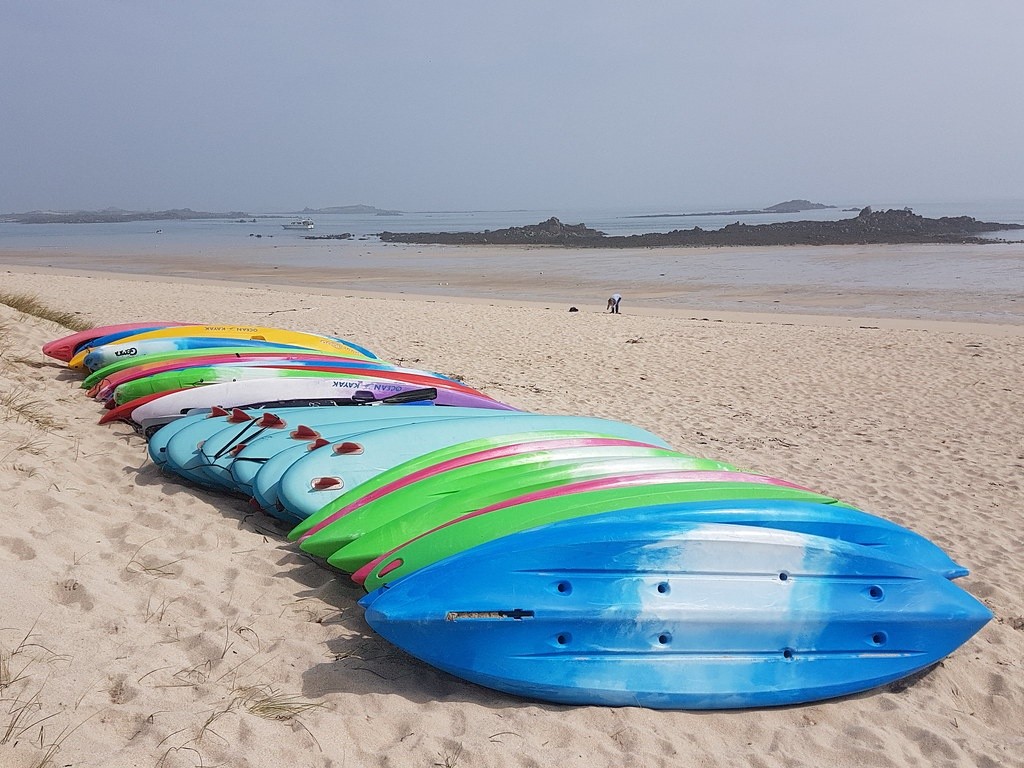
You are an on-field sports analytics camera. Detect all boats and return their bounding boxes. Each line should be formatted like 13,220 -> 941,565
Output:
281,218 -> 314,230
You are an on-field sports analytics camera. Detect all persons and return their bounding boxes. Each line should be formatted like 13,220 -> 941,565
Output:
607,294 -> 622,314
